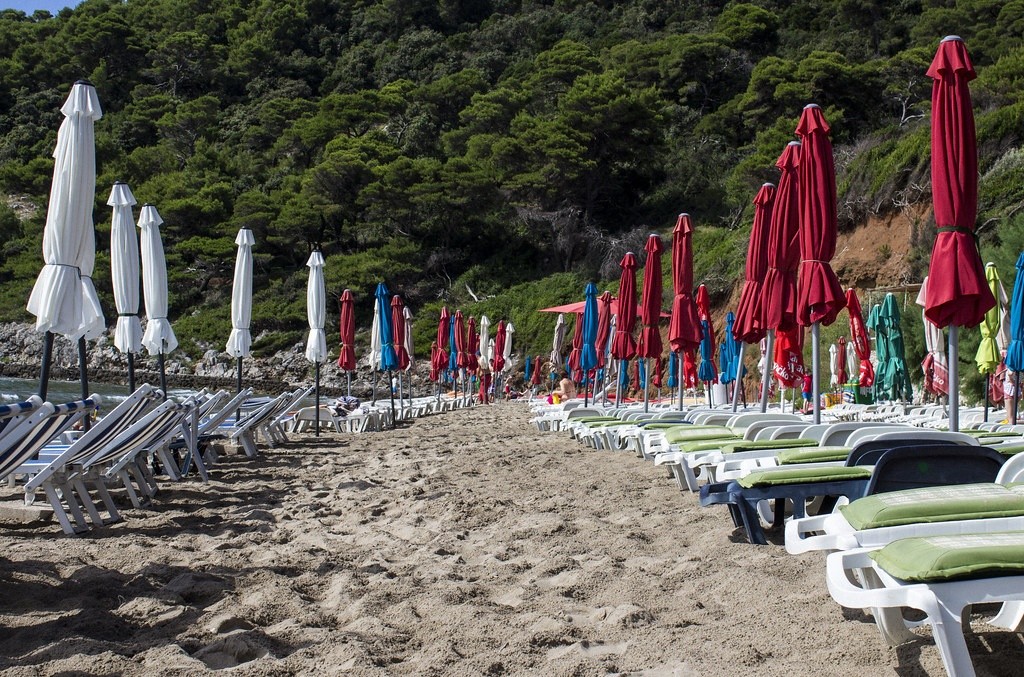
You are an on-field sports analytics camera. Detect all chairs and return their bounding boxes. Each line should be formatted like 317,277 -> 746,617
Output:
0,380 -> 1024,677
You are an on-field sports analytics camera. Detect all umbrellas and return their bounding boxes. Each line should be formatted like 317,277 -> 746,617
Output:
305,240 -> 328,438
565,213 -> 748,412
731,104 -> 847,424
338,289 -> 356,396
369,283 -> 416,429
226,224 -> 256,443
537,291 -> 673,318
106,180 -> 178,399
430,306 -> 516,406
550,313 -> 567,394
524,354 -> 541,395
25,80 -> 105,435
828,251 -> 1024,423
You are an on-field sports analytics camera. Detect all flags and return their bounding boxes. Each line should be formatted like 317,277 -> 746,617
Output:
925,34 -> 999,432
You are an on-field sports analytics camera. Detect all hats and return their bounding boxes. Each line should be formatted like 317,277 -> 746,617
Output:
804,367 -> 812,372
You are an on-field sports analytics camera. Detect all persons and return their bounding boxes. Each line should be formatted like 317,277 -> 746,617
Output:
801,368 -> 813,414
546,370 -> 576,404
1003,367 -> 1024,423
505,374 -> 530,402
